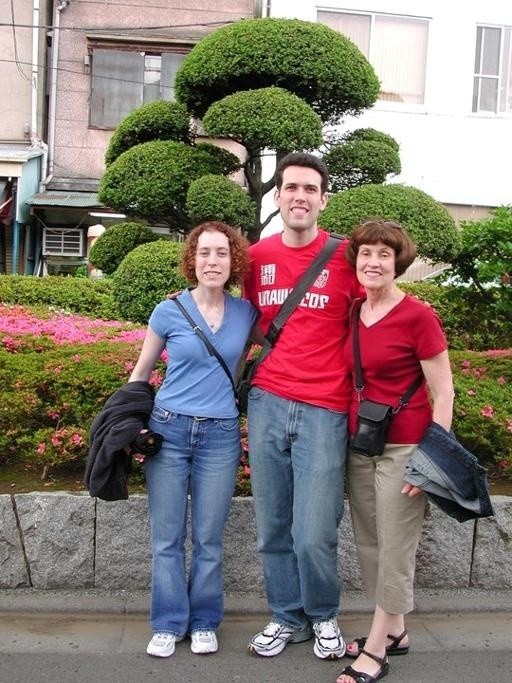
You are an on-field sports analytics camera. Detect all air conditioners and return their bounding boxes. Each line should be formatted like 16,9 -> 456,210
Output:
42,227 -> 87,257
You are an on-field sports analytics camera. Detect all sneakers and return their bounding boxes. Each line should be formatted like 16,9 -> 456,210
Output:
189,627 -> 219,655
246,618 -> 313,658
146,631 -> 186,659
312,615 -> 347,660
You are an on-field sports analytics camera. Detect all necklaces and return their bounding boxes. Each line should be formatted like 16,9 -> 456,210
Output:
197,296 -> 225,332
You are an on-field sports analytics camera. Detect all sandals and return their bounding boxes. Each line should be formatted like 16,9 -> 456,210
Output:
346,628 -> 410,658
337,650 -> 391,683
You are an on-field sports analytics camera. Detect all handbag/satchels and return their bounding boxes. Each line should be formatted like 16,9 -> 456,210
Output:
234,356 -> 261,416
349,397 -> 395,459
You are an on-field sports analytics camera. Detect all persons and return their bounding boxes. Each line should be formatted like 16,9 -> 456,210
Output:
334,218 -> 457,681
166,150 -> 434,663
122,218 -> 261,659
